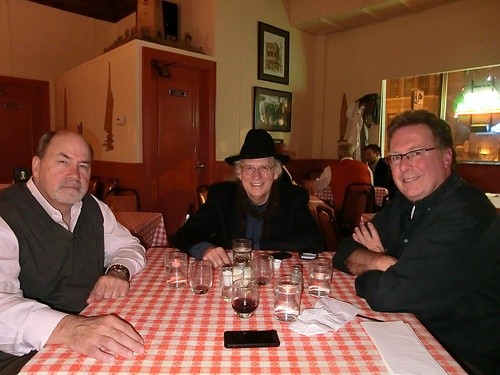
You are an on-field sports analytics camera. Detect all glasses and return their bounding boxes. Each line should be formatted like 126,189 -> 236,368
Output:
239,163 -> 272,176
383,147 -> 439,163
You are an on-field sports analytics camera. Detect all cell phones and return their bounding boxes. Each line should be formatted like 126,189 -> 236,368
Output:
224,329 -> 280,348
262,252 -> 292,260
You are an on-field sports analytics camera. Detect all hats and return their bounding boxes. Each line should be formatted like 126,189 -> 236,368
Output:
224,127 -> 291,167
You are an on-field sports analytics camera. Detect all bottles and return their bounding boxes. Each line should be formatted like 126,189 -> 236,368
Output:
222,263 -> 234,299
289,264 -> 304,290
233,257 -> 252,287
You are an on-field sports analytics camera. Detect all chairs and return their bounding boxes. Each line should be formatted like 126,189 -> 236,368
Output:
104,187 -> 140,212
316,183 -> 375,251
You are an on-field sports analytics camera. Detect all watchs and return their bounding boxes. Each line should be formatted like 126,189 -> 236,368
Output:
106,264 -> 130,281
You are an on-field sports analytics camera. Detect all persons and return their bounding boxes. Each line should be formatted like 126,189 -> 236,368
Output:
0,128 -> 146,366
172,129 -> 327,268
332,110 -> 500,375
315,143 -> 394,211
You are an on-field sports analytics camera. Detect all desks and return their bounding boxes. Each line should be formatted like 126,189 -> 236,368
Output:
113,212 -> 168,249
17,248 -> 469,375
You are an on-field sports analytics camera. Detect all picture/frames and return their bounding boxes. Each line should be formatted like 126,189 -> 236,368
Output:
252,86 -> 292,132
258,21 -> 290,85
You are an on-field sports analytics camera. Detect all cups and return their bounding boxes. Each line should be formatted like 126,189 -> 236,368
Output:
309,259 -> 333,298
219,238 -> 282,318
273,276 -> 301,322
190,260 -> 213,294
164,251 -> 188,290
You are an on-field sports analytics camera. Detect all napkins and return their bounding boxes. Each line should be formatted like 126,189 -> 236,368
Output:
360,320 -> 449,375
288,295 -> 361,336
